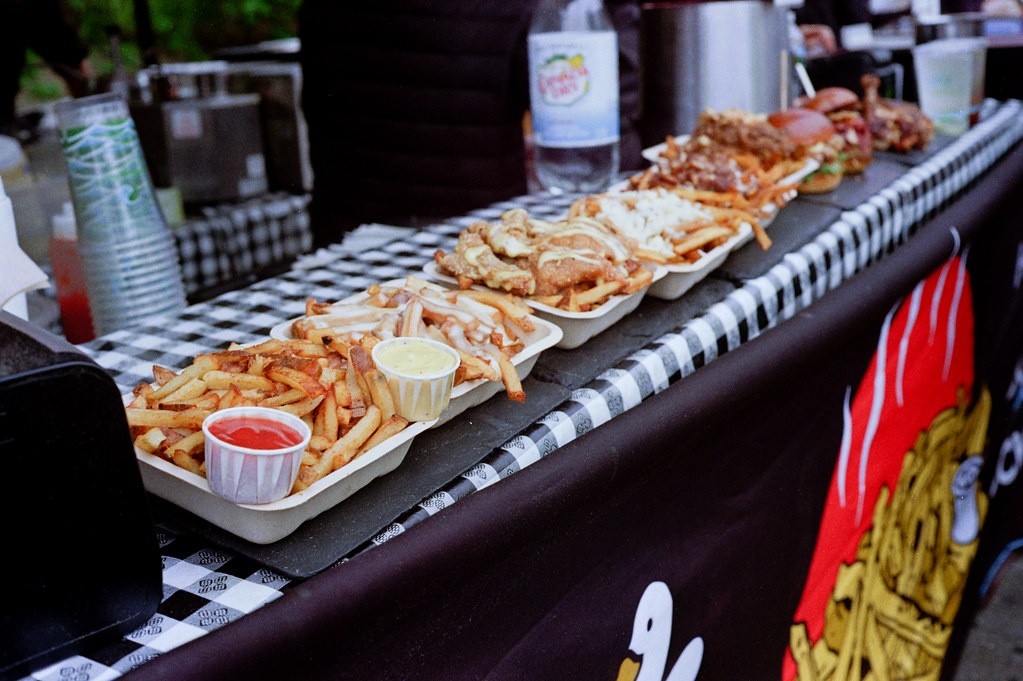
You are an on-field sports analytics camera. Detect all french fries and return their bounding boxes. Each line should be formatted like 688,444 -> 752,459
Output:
291,272 -> 535,402
433,135 -> 802,314
125,336 -> 408,498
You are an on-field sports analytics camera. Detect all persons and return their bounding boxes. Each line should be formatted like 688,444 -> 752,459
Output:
0,0 -> 96,130
297,0 -> 536,252
796,0 -> 881,102
639,0 -> 838,171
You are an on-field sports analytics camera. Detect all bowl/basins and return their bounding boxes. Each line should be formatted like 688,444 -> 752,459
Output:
371,336 -> 460,421
202,404 -> 312,506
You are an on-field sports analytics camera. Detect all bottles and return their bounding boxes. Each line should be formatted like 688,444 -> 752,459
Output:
526,0 -> 619,197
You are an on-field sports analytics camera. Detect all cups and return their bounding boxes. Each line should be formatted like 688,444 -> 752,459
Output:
942,36 -> 989,127
51,92 -> 189,336
911,43 -> 971,134
673,0 -> 782,138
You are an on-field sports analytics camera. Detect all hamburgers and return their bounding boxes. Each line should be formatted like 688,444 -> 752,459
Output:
766,87 -> 874,193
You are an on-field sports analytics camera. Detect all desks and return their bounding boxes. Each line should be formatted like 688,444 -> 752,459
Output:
0,100 -> 1023,681
25,190 -> 313,336
